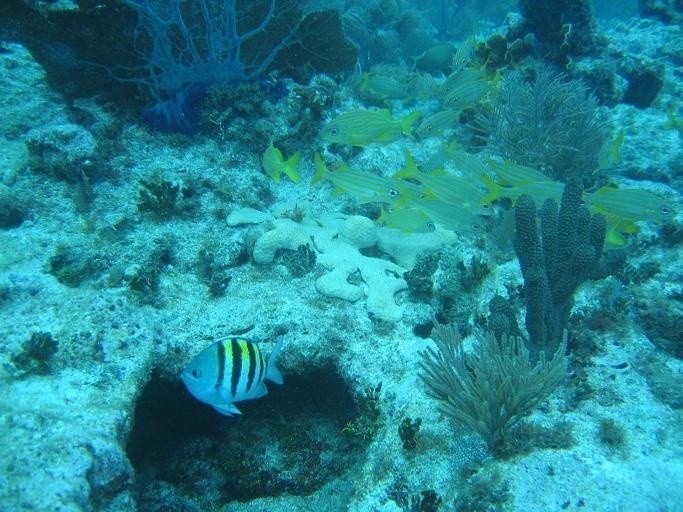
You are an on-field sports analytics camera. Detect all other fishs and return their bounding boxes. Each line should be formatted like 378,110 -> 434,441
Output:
308,1 -> 564,246
